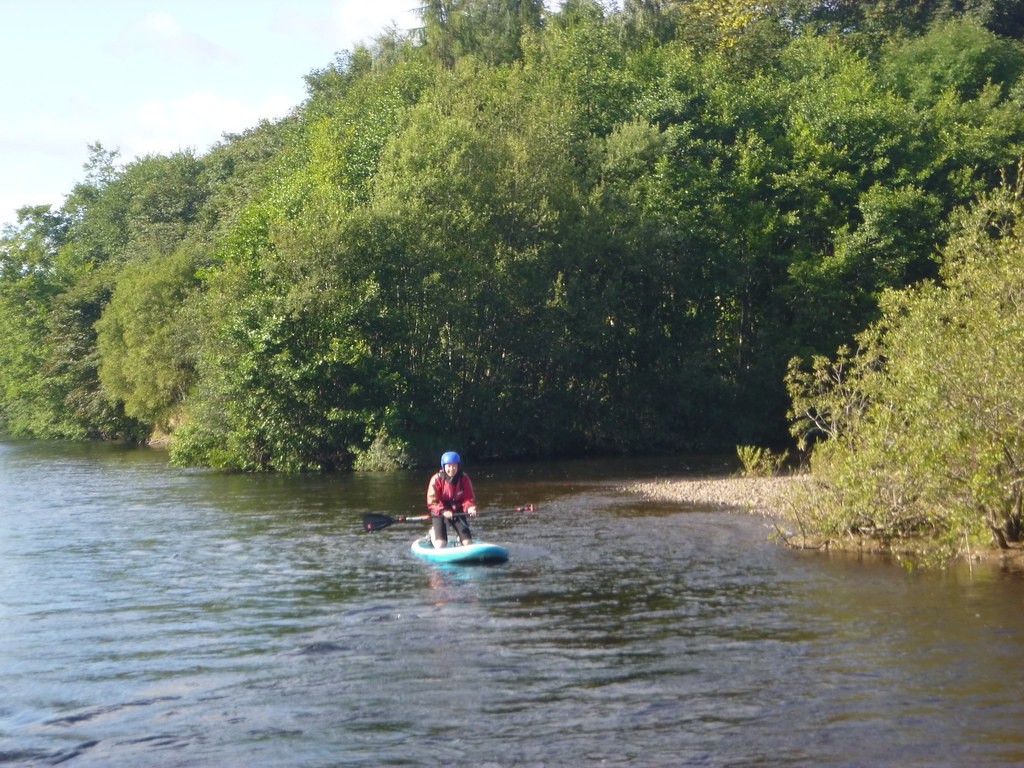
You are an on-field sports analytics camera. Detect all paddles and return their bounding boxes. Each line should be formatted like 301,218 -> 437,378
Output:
359,501 -> 535,535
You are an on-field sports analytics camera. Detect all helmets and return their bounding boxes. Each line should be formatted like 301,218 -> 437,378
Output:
441,452 -> 461,472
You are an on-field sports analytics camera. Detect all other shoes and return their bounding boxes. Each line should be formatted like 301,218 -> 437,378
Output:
454,535 -> 461,547
426,529 -> 432,545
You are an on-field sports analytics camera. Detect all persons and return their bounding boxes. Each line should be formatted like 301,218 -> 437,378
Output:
426,451 -> 478,549
